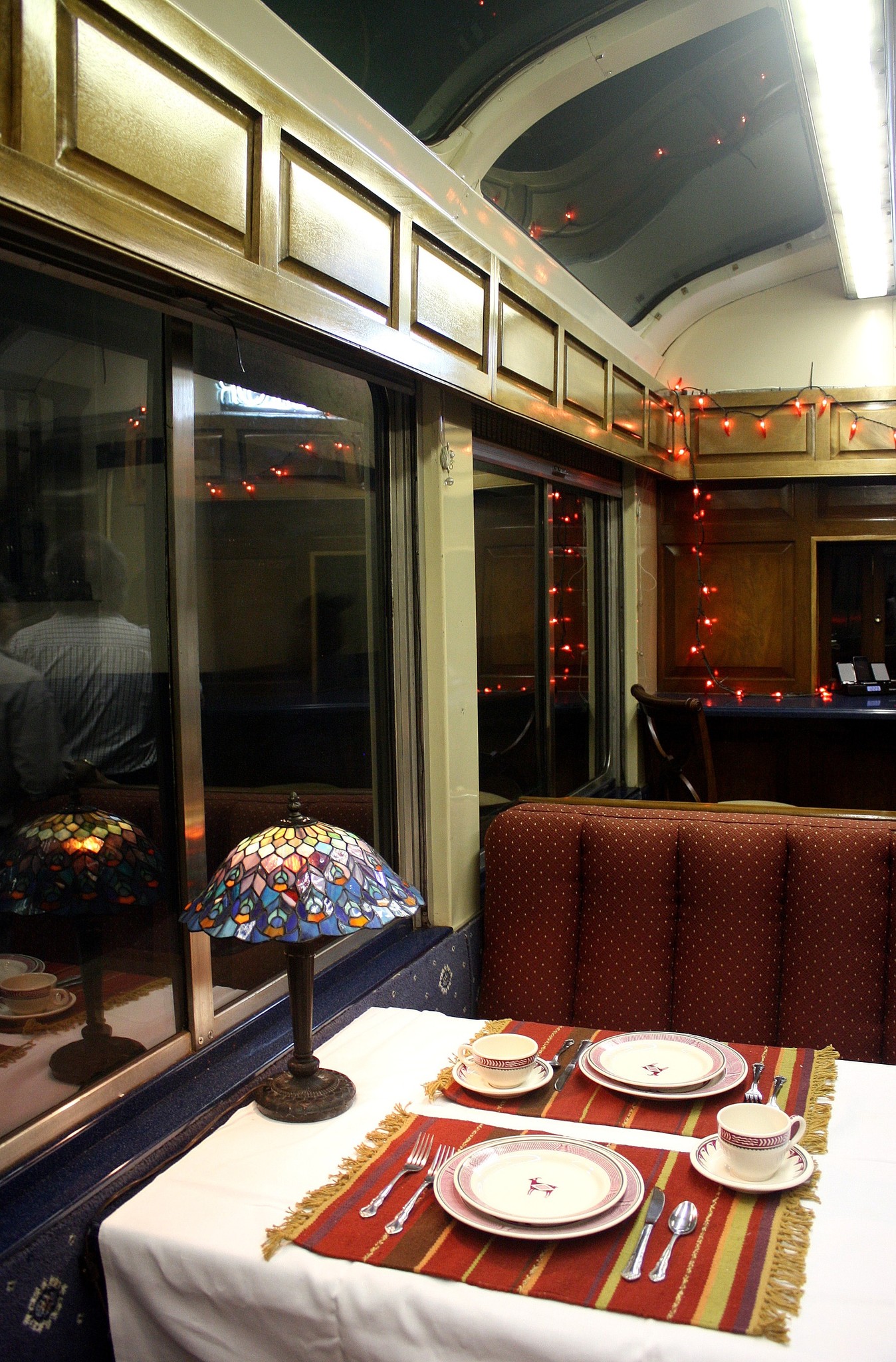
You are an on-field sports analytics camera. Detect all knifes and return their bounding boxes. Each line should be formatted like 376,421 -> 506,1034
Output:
620,1186 -> 665,1279
554,1039 -> 592,1092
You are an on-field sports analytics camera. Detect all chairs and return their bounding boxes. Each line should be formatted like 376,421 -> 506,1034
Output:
629,683 -> 717,804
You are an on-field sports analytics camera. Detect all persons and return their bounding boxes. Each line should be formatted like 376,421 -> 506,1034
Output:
0,654 -> 69,794
9,530 -> 158,787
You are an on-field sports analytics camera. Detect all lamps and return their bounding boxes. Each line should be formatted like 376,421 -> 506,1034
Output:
175,790 -> 427,1122
0,755 -> 174,1089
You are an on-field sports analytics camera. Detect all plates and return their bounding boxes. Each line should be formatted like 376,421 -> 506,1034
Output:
579,1031 -> 749,1101
0,954 -> 47,973
1,991 -> 78,1022
690,1134 -> 818,1196
452,1055 -> 553,1098
433,1134 -> 645,1240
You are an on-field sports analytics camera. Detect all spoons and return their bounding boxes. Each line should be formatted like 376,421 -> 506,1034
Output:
546,1039 -> 576,1066
647,1201 -> 698,1282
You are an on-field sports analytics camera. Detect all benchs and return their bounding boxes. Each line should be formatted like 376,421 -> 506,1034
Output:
475,797 -> 896,1066
0,781 -> 377,991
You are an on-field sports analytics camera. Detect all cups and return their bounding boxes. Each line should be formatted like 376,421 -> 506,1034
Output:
0,973 -> 70,1014
716,1103 -> 809,1182
458,1033 -> 539,1089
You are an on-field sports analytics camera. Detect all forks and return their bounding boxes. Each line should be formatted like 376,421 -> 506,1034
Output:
745,1063 -> 765,1102
358,1131 -> 435,1217
385,1144 -> 456,1235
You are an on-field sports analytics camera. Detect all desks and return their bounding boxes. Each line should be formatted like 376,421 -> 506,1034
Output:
0,954 -> 247,1142
89,1007 -> 896,1362
636,692 -> 896,811
